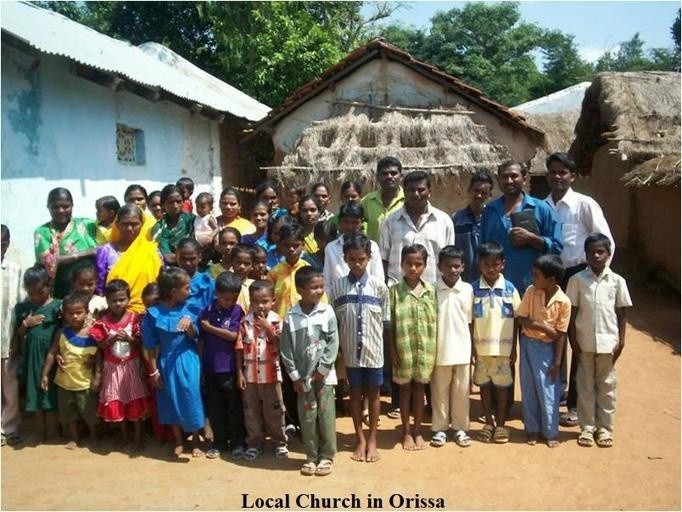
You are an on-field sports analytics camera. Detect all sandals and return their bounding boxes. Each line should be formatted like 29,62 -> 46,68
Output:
491,425 -> 510,443
1,434 -> 23,445
596,428 -> 612,446
453,431 -> 471,446
476,424 -> 493,442
301,459 -> 333,475
430,432 -> 446,446
578,431 -> 594,445
207,446 -> 288,461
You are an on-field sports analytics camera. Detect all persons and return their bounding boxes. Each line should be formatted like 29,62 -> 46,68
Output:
3,150 -> 638,476
